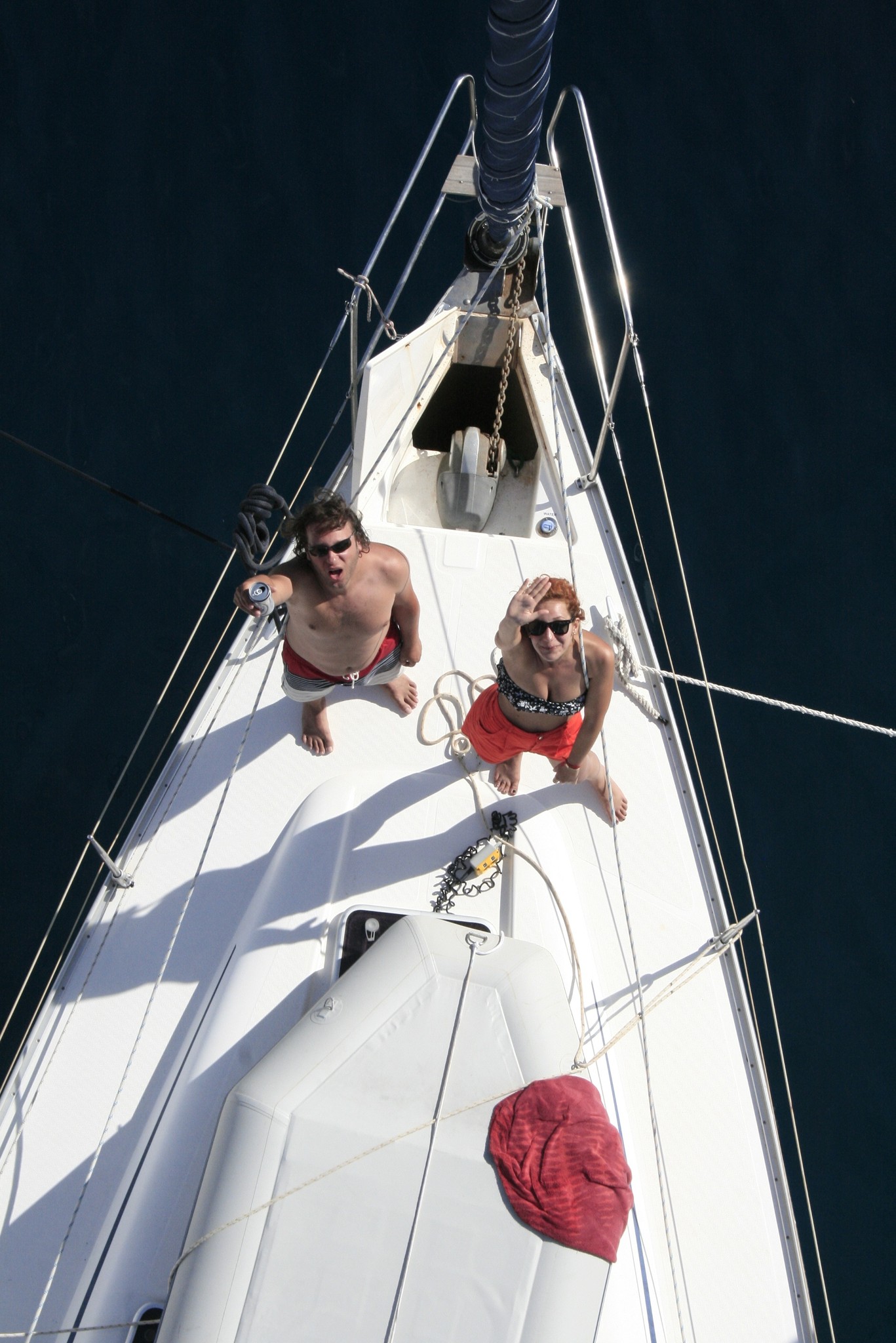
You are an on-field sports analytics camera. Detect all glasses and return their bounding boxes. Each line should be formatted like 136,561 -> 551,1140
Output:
306,530 -> 356,558
527,616 -> 574,636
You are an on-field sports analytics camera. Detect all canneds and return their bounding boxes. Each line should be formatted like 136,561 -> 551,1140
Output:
248,582 -> 275,616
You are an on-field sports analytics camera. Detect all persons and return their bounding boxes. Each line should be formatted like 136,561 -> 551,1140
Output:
234,492 -> 423,755
458,574 -> 628,826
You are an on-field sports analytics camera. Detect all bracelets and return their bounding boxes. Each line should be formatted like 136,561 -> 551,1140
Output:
562,759 -> 581,770
